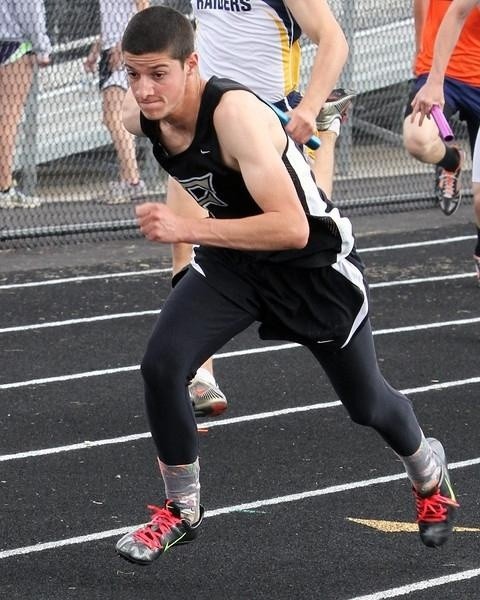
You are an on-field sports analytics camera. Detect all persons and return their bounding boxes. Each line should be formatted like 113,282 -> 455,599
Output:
82,0 -> 152,208
409,0 -> 480,262
114,5 -> 462,566
1,1 -> 55,213
400,0 -> 480,286
163,0 -> 360,420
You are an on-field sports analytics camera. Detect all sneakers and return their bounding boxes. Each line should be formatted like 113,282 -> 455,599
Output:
115,496 -> 206,566
184,373 -> 228,417
409,436 -> 459,550
95,179 -> 149,205
435,143 -> 466,215
1,187 -> 42,208
